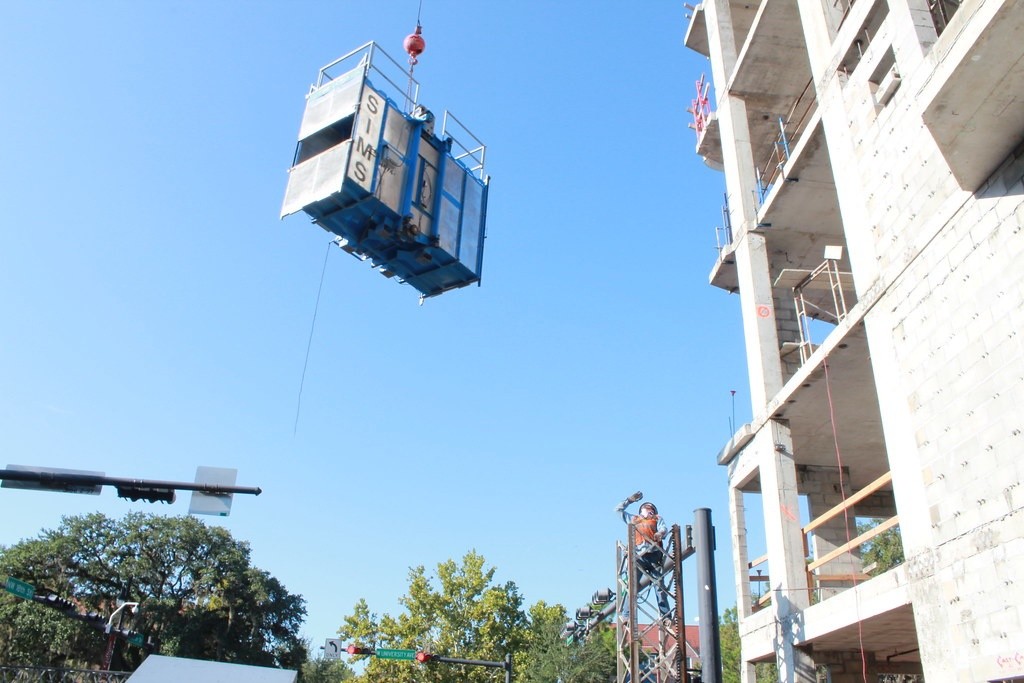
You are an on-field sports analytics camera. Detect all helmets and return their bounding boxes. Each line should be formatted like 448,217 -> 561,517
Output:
639,502 -> 658,516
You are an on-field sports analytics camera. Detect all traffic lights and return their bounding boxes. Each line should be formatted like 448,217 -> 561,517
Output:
416,652 -> 440,665
347,645 -> 371,656
119,628 -> 138,639
86,612 -> 107,625
592,588 -> 613,605
559,620 -> 578,639
576,605 -> 592,621
45,593 -> 76,612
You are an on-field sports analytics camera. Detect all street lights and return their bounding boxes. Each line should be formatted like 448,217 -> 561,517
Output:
105,573 -> 133,683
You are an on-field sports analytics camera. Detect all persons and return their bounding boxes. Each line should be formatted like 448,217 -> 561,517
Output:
615,488 -> 674,629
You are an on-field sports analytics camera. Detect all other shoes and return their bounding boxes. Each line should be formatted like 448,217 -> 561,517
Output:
663,619 -> 675,627
621,619 -> 627,626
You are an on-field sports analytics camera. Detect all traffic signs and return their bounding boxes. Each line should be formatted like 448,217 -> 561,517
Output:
566,634 -> 574,647
127,631 -> 144,646
376,648 -> 416,661
4,576 -> 35,601
